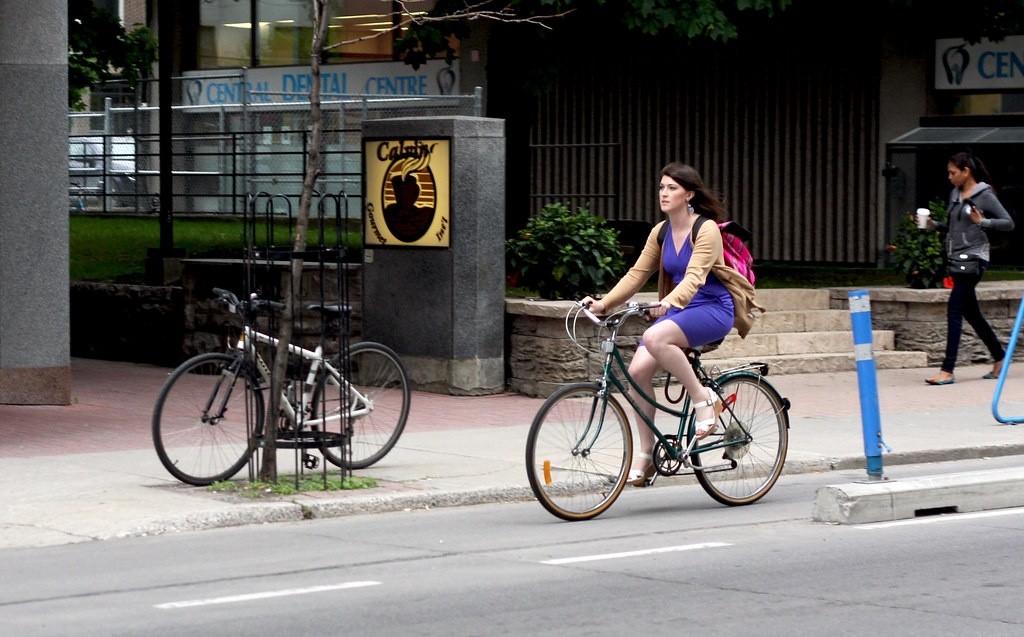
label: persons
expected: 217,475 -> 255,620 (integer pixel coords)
913,149 -> 1011,385
578,160 -> 736,487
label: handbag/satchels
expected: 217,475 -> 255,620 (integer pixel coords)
947,254 -> 981,279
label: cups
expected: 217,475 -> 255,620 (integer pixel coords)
917,208 -> 929,229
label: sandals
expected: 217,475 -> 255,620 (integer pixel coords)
692,387 -> 723,440
625,453 -> 656,485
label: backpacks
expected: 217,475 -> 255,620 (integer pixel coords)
656,214 -> 756,293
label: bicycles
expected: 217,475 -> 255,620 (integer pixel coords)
521,293 -> 793,525
72,181 -> 96,211
150,285 -> 413,488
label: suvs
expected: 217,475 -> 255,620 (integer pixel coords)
67,134 -> 137,209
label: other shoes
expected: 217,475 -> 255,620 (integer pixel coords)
924,371 -> 956,384
982,359 -> 1012,379
